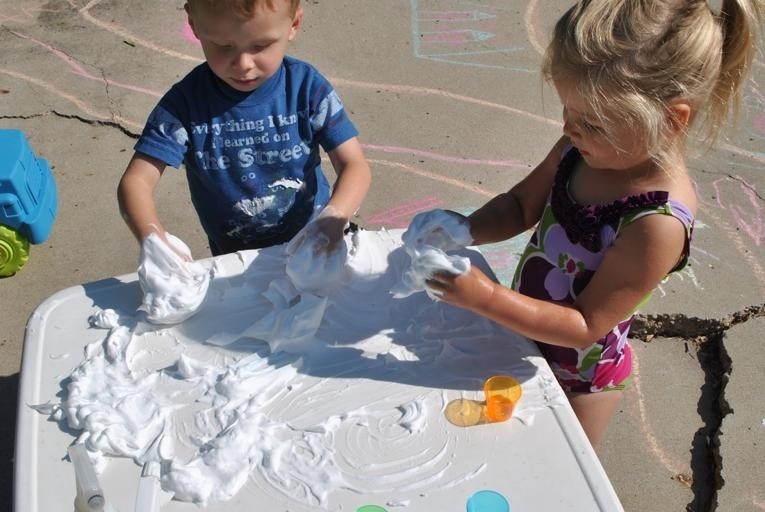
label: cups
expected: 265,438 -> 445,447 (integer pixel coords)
485,375 -> 521,422
466,490 -> 509,512
445,399 -> 489,428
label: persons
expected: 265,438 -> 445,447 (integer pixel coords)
402,0 -> 765,448
116,0 -> 373,310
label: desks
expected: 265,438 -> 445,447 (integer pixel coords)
11,228 -> 626,511
